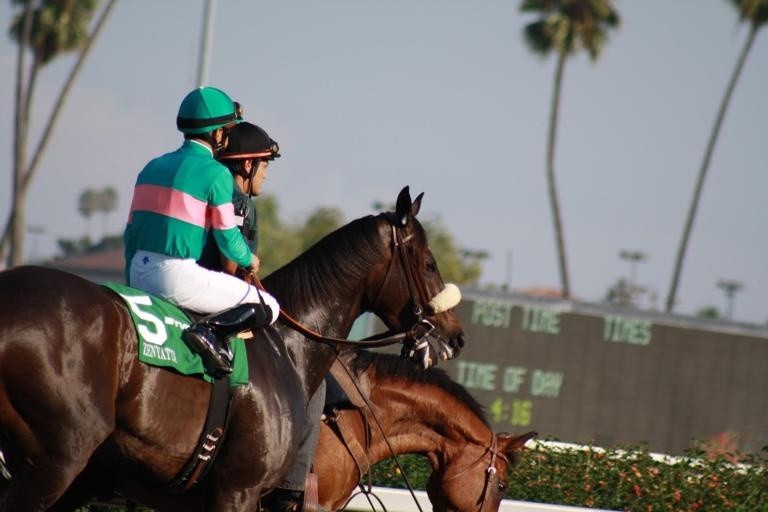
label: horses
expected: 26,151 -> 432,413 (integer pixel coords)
255,346 -> 539,512
0,185 -> 467,512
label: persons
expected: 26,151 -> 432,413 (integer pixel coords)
197,122 -> 280,270
120,86 -> 280,378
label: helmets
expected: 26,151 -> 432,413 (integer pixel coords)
221,122 -> 281,161
175,87 -> 245,134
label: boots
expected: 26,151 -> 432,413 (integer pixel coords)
183,302 -> 270,375
